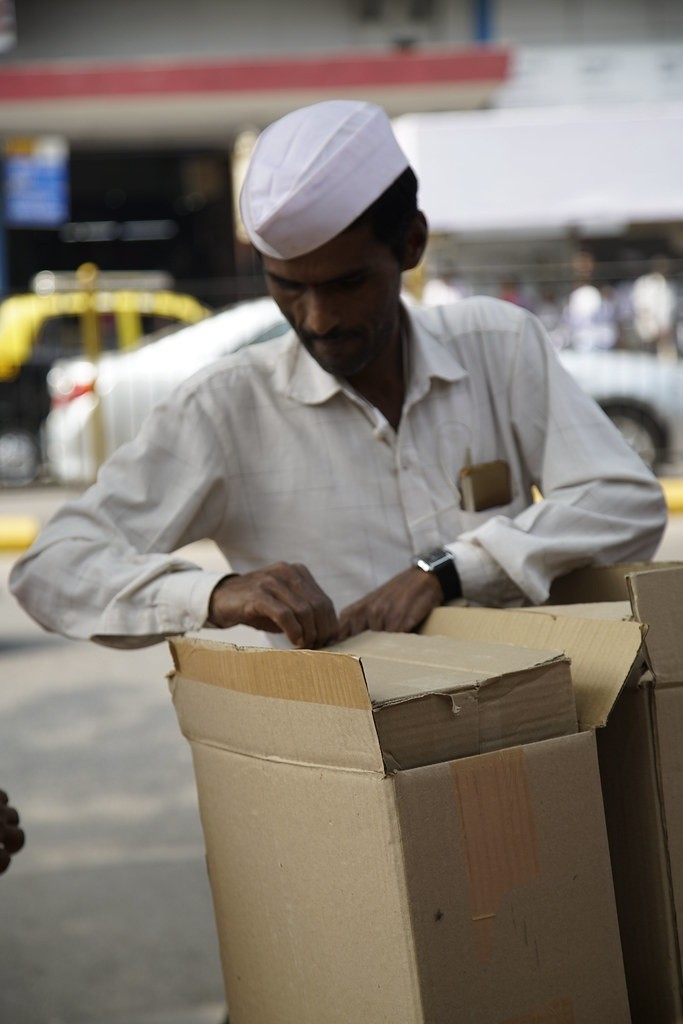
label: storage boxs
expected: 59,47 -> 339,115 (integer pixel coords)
164,559 -> 683,1024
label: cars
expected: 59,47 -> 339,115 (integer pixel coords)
45,295 -> 683,494
0,292 -> 214,489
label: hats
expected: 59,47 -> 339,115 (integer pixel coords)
240,99 -> 411,262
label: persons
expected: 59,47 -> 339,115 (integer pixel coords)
16,98 -> 668,653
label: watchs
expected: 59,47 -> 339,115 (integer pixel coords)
410,545 -> 463,607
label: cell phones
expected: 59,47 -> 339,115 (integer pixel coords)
460,458 -> 512,512
